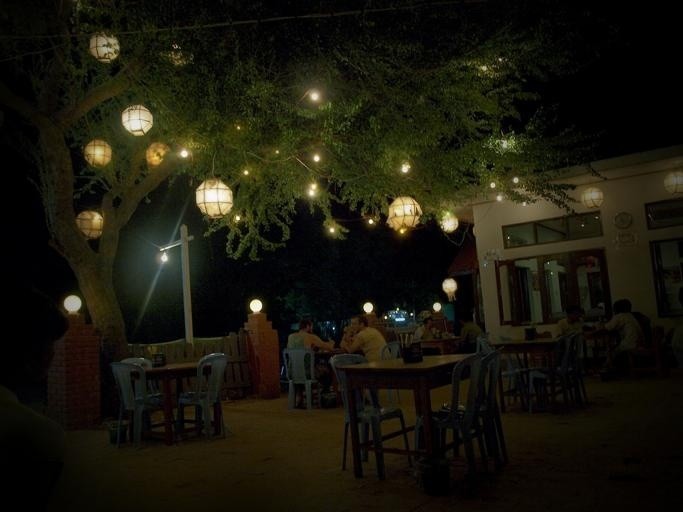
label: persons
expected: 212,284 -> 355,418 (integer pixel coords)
412,317 -> 437,342
338,326 -> 357,349
552,297 -> 653,381
456,310 -> 484,353
281,318 -> 335,409
342,315 -> 392,363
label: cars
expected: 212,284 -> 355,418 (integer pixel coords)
383,313 -> 408,328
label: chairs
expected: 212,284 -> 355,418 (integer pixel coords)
331,353 -> 412,480
412,349 -> 508,481
178,353 -> 228,441
484,325 -> 611,416
597,324 -> 683,381
283,325 -> 484,409
110,353 -> 164,448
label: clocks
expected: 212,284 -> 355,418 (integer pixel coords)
613,210 -> 634,229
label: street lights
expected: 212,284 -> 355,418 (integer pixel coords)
157,225 -> 195,343
